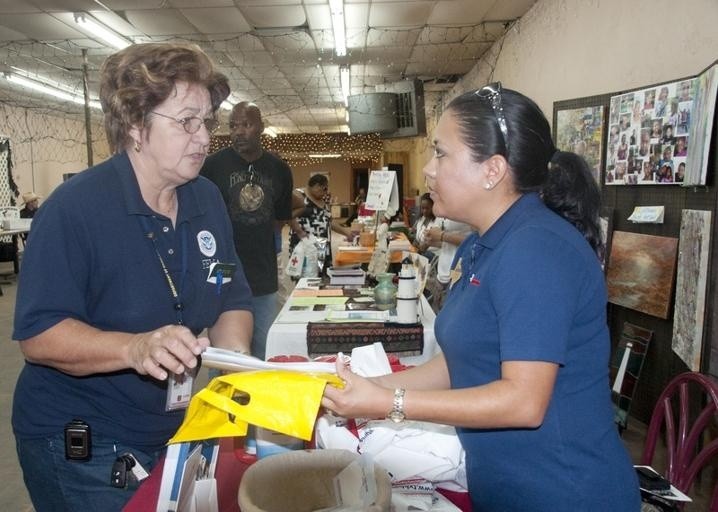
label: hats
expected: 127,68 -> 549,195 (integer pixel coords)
21,192 -> 43,206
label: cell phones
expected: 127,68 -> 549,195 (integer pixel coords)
65,422 -> 92,463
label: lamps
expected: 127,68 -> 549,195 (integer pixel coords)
327,0 -> 352,139
0,8 -> 280,139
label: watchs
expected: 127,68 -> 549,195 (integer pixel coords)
389,387 -> 405,423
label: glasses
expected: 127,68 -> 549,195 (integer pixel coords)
147,110 -> 220,134
475,81 -> 510,162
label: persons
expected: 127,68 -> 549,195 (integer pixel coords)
8,32 -> 253,512
322,80 -> 643,512
274,193 -> 305,253
288,160 -> 355,286
607,82 -> 695,183
424,215 -> 473,316
413,191 -> 439,263
20,191 -> 42,219
200,102 -> 294,381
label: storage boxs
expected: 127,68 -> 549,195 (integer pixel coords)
3,218 -> 32,230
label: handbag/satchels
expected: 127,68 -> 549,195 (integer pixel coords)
291,239 -> 318,280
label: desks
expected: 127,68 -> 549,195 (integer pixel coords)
0,229 -> 31,273
334,232 -> 417,268
330,202 -> 356,218
125,355 -> 473,512
265,277 -> 440,367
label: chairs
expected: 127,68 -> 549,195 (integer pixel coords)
633,370 -> 718,512
